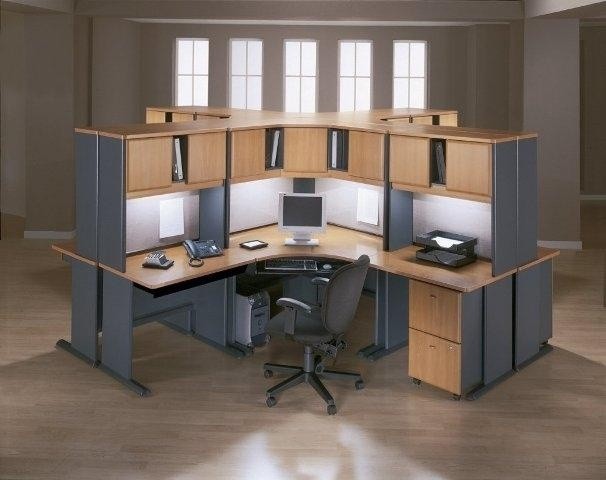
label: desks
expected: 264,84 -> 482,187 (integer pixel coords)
52,223 -> 561,402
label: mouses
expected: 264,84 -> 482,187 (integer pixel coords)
323,263 -> 332,270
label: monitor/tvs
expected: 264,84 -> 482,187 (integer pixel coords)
278,192 -> 326,246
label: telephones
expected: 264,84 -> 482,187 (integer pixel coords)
183,239 -> 225,267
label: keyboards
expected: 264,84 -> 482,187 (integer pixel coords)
265,259 -> 318,271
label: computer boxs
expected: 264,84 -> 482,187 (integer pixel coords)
235,281 -> 271,347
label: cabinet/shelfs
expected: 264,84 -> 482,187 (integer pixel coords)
75,107 -> 539,278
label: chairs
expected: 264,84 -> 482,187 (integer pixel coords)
262,254 -> 370,415
